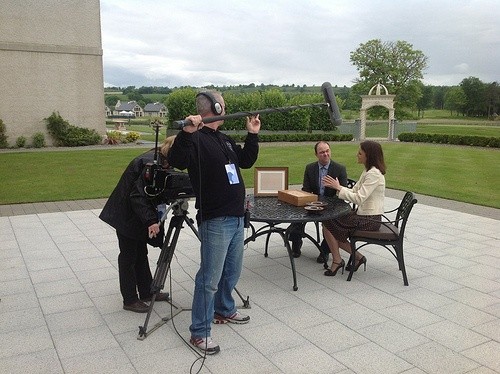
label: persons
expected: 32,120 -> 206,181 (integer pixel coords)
98,135 -> 177,313
291,141 -> 348,262
167,90 -> 260,354
323,141 -> 386,276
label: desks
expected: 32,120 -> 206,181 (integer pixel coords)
242,193 -> 351,292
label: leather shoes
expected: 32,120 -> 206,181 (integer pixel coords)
291,240 -> 302,257
141,291 -> 169,302
317,243 -> 329,262
123,300 -> 152,313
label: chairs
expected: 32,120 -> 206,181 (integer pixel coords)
285,179 -> 418,286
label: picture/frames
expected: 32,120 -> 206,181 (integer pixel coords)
253,167 -> 288,198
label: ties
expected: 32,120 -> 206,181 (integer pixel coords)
320,168 -> 327,195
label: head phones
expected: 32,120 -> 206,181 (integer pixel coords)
195,91 -> 222,116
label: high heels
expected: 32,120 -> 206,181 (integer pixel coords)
346,256 -> 366,272
324,259 -> 345,276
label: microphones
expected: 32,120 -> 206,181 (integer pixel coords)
321,81 -> 342,127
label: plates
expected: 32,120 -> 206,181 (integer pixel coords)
304,206 -> 324,210
306,201 -> 328,206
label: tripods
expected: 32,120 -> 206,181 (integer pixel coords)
136,198 -> 251,340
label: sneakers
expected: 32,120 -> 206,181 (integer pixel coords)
212,311 -> 250,325
189,336 -> 220,354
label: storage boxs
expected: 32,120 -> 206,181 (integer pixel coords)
277,190 -> 318,207
